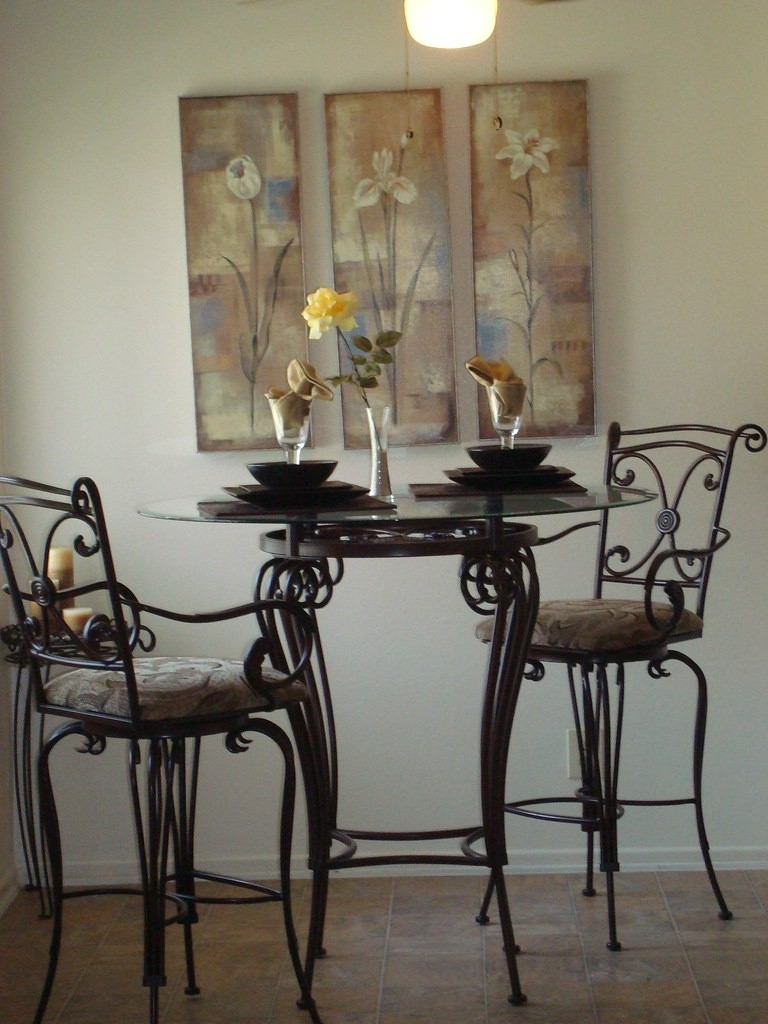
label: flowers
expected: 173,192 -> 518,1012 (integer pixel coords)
300,285 -> 402,406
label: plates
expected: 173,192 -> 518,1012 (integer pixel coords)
443,464 -> 577,488
222,481 -> 370,510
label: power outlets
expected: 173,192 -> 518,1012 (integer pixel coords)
567,728 -> 605,781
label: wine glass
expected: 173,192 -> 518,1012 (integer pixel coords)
485,387 -> 528,452
270,398 -> 312,465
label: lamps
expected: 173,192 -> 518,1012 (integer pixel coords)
403,0 -> 497,49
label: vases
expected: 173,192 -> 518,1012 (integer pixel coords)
364,408 -> 396,502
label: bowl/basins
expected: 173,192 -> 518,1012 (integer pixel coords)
245,459 -> 339,489
465,443 -> 552,472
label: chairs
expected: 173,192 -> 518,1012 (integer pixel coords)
0,473 -> 328,1024
475,419 -> 768,950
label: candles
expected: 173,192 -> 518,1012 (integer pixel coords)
27,577 -> 61,631
47,547 -> 76,607
63,606 -> 93,635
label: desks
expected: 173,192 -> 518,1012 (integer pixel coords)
4,620 -> 129,916
138,487 -> 658,1012
